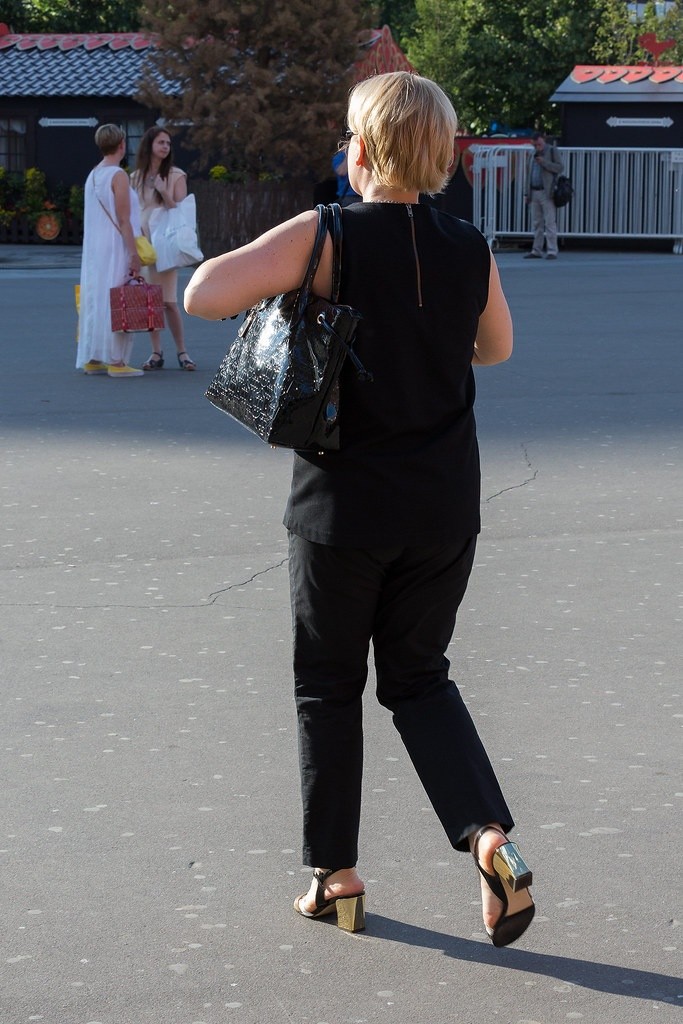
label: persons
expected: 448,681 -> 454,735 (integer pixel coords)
75,125 -> 196,376
524,134 -> 565,259
184,71 -> 537,947
332,150 -> 363,208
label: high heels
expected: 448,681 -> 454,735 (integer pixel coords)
472,820 -> 537,948
294,866 -> 366,934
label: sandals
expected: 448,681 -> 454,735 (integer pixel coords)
176,350 -> 197,370
140,349 -> 165,371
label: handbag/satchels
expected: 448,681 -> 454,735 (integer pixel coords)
552,175 -> 572,208
110,276 -> 165,331
204,203 -> 360,453
130,235 -> 158,267
147,192 -> 204,273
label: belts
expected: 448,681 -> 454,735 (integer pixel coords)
528,185 -> 543,190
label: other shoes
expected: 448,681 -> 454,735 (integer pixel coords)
545,254 -> 557,259
523,252 -> 542,258
84,361 -> 107,375
108,364 -> 144,377
